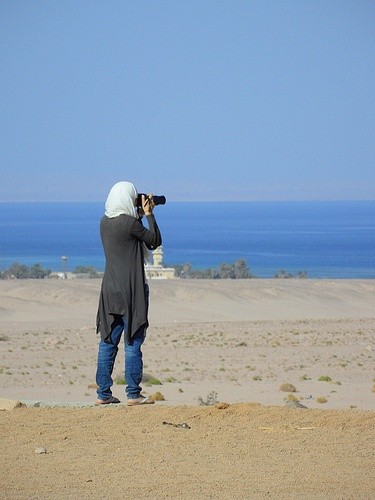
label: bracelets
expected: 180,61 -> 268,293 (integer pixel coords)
144,210 -> 152,213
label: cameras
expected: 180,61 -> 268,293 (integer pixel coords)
138,194 -> 166,207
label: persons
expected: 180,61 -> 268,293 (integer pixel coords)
92,180 -> 163,405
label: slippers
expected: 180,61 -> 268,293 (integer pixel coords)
127,397 -> 155,406
95,396 -> 120,406
